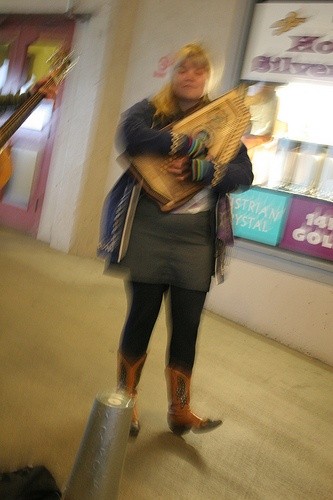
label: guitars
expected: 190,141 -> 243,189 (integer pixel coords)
0,45 -> 79,193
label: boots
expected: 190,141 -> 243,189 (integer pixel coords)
115,350 -> 147,436
163,366 -> 223,436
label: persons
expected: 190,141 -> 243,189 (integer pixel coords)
0,71 -> 61,112
93,44 -> 254,438
